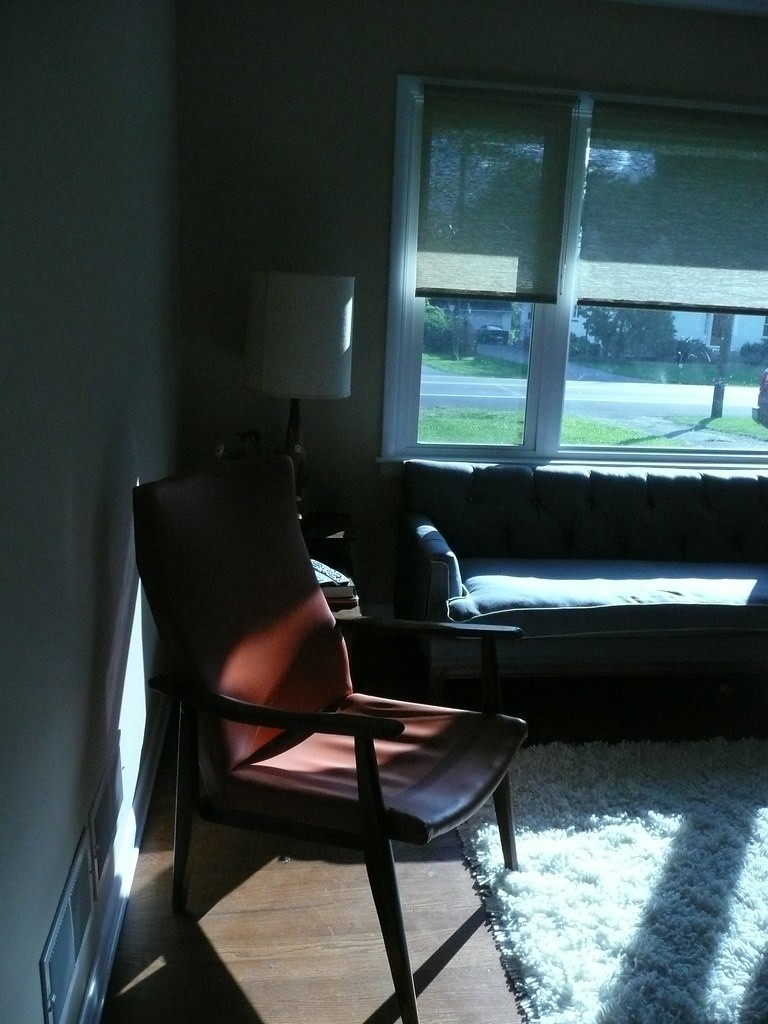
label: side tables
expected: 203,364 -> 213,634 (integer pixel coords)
301,511 -> 352,569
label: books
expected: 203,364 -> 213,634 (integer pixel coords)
309,557 -> 359,608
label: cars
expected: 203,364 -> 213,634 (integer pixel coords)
477,325 -> 509,345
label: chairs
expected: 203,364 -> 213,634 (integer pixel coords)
131,452 -> 527,1024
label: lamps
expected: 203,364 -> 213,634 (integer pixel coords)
241,269 -> 356,522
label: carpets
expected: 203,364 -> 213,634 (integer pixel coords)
456,734 -> 768,1024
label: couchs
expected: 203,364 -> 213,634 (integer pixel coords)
389,460 -> 768,707
